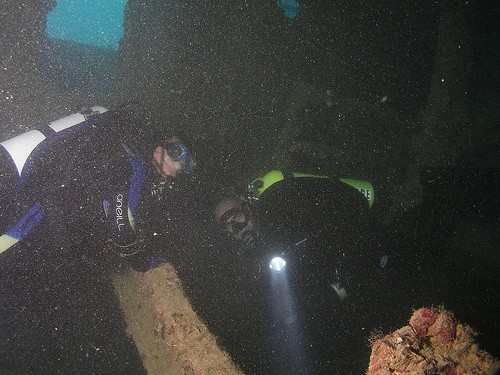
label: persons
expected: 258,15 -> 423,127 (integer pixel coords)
20,93 -> 201,274
208,159 -> 500,340
